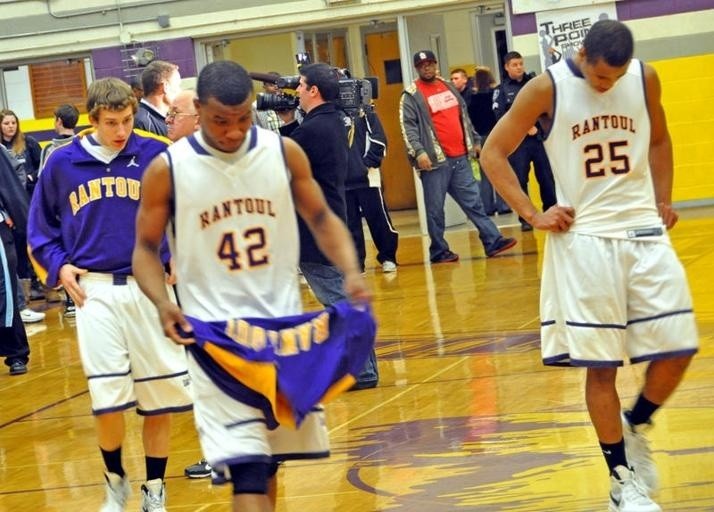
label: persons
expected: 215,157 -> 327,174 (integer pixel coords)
162,89 -> 230,485
128,62 -> 374,512
489,20 -> 700,512
25,78 -> 194,512
1,49 -> 559,373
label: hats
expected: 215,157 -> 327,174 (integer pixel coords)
414,50 -> 437,65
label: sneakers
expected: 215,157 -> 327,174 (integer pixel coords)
608,466 -> 664,511
382,260 -> 396,272
207,463 -> 232,484
9,360 -> 27,375
619,407 -> 661,491
488,237 -> 516,257
141,479 -> 169,512
522,222 -> 533,232
185,458 -> 210,478
97,478 -> 132,512
14,288 -> 76,323
431,249 -> 458,262
